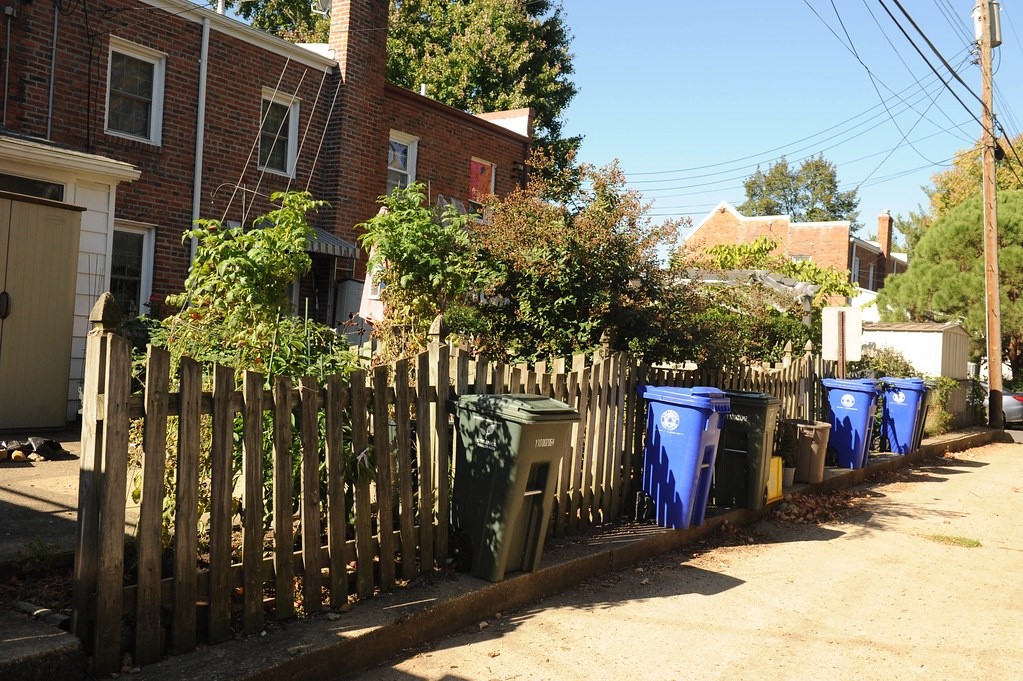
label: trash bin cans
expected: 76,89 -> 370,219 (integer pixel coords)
634,381 -> 732,530
819,378 -> 882,469
880,377 -> 933,454
783,419 -> 832,484
447,392 -> 582,582
715,389 -> 782,511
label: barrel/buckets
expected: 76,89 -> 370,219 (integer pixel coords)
783,467 -> 796,487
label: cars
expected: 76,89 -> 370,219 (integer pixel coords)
966,379 -> 1023,430
